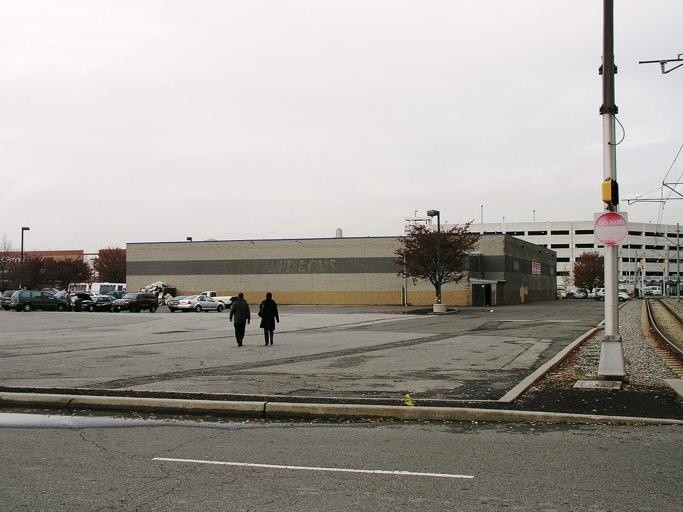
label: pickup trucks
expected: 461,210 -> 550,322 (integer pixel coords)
198,289 -> 238,309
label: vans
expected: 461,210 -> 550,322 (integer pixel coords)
643,285 -> 661,295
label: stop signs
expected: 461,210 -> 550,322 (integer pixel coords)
593,210 -> 629,246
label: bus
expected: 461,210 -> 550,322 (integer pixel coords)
66,281 -> 90,293
89,282 -> 125,293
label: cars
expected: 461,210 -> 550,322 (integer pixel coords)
0,287 -> 159,313
166,294 -> 188,313
555,284 -> 631,302
177,295 -> 226,313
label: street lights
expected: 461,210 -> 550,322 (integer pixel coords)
426,210 -> 446,313
19,226 -> 30,289
414,210 -> 417,224
186,237 -> 192,242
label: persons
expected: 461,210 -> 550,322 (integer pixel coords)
230,293 -> 250,346
260,292 -> 279,345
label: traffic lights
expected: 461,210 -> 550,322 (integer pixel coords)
601,177 -> 619,205
639,260 -> 644,268
662,262 -> 668,268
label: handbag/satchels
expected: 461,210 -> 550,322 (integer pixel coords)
258,309 -> 264,317
